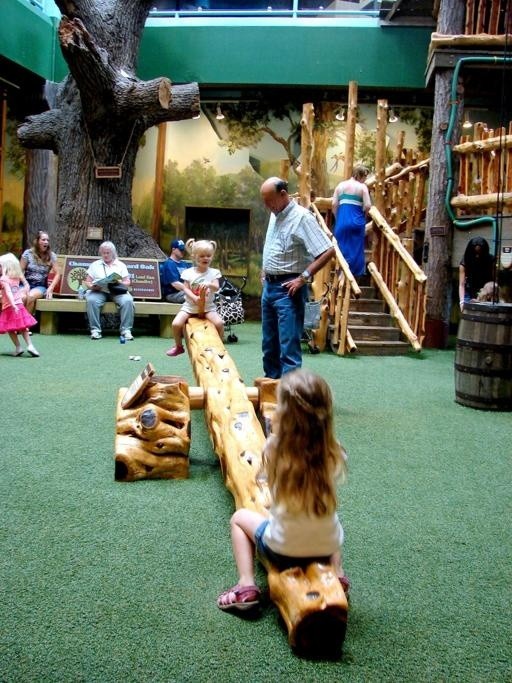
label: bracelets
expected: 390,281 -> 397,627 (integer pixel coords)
301,268 -> 311,280
459,299 -> 465,304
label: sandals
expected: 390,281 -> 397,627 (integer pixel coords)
334,577 -> 355,601
218,584 -> 267,610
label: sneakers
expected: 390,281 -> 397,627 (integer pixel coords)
166,345 -> 185,356
120,330 -> 135,341
25,346 -> 40,358
14,345 -> 24,357
90,332 -> 103,339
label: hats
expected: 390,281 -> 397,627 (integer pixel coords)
171,239 -> 186,250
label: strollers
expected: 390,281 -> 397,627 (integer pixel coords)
213,276 -> 249,345
295,281 -> 330,354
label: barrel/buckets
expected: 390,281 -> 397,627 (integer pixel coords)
453,301 -> 512,413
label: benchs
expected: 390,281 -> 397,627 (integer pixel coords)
35,299 -> 181,339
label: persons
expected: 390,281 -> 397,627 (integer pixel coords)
217,366 -> 350,618
456,234 -> 498,311
166,236 -> 225,356
83,239 -> 135,342
330,164 -> 370,281
1,251 -> 40,357
161,238 -> 187,303
259,175 -> 336,376
19,229 -> 61,335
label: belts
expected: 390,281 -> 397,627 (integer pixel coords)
264,273 -> 301,283
